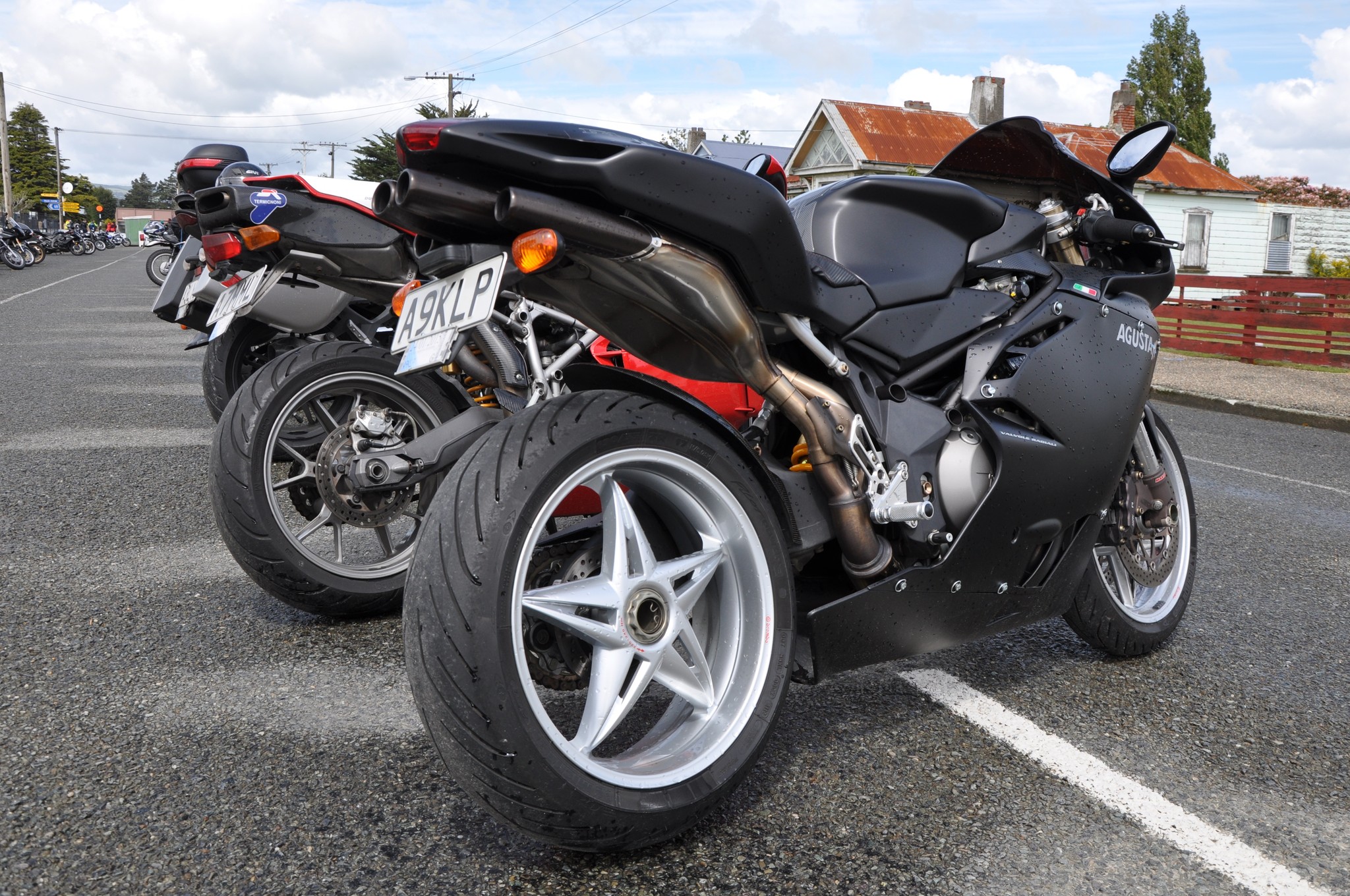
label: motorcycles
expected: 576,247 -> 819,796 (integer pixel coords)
144,220 -> 179,286
174,154 -> 790,618
146,195 -> 396,460
373,117 -> 1202,851
0,211 -> 131,270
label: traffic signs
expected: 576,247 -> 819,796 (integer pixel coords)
41,193 -> 79,213
